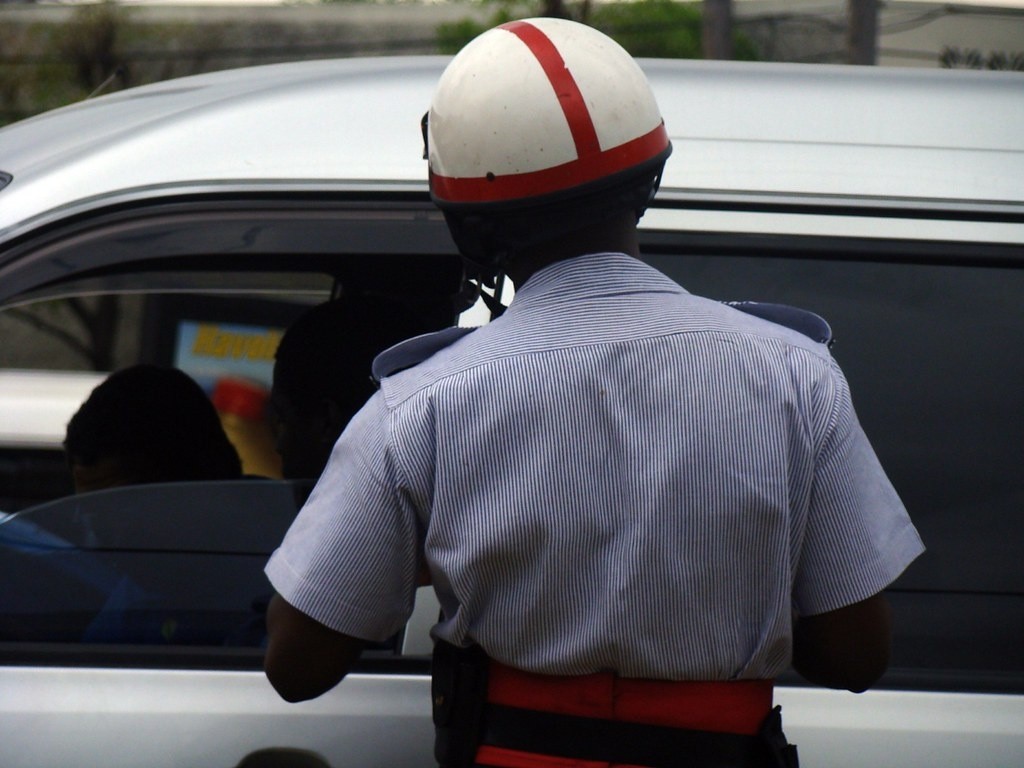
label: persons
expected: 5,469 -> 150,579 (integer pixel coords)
63,295 -> 431,647
262,16 -> 926,767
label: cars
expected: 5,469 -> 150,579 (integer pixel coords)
1,53 -> 1024,767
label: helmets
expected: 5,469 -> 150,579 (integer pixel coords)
421,18 -> 672,263
